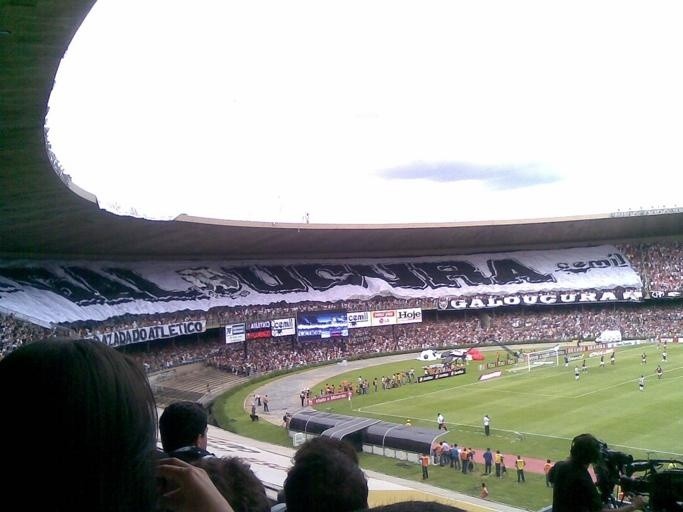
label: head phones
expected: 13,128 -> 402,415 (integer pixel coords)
570,433 -> 587,456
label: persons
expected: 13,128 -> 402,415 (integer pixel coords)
543,434 -> 683,511
419,413 -> 526,498
1,338 -> 464,510
202,239 -> 683,392
1,309 -> 201,359
